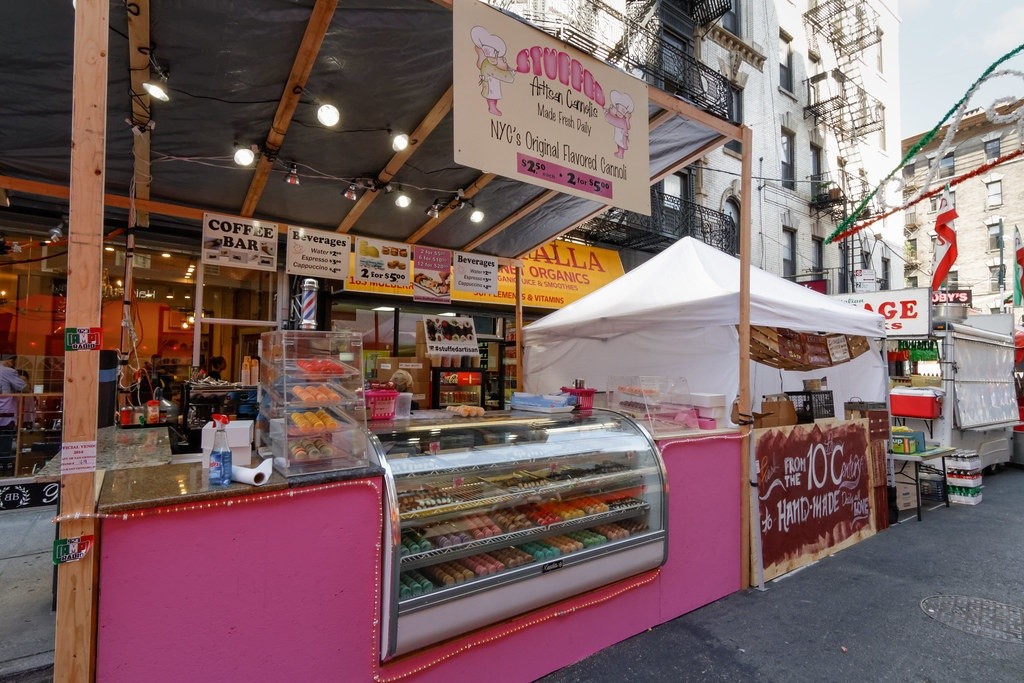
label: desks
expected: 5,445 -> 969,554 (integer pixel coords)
179,384 -> 257,436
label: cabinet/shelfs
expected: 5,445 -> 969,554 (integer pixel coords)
387,436 -> 664,609
259,333 -> 370,478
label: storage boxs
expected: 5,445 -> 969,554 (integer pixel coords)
844,399 -> 889,439
201,420 -> 254,448
947,493 -> 982,505
691,392 -> 727,430
804,378 -> 828,394
561,385 -> 596,410
365,321 -> 431,420
893,434 -> 915,454
754,399 -> 797,429
946,472 -> 982,489
890,386 -> 943,419
920,477 -> 945,501
203,445 -> 251,468
944,454 -> 981,471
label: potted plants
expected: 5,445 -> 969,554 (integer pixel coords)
811,181 -> 841,205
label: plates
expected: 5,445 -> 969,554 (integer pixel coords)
414,281 -> 450,297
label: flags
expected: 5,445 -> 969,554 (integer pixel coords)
930,184 -> 962,291
1012,224 -> 1024,309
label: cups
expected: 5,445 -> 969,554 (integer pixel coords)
431,353 -> 442,366
251,360 -> 258,385
242,362 -> 250,386
462,356 -> 472,368
229,415 -> 237,419
442,354 -> 451,367
451,355 -> 462,368
472,355 -> 481,368
244,356 -> 251,365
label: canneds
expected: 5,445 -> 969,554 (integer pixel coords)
440,392 -> 479,404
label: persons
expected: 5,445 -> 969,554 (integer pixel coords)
0,348 -> 36,478
134,352 -> 174,405
390,370 -> 420,410
204,356 -> 226,420
254,342 -> 348,448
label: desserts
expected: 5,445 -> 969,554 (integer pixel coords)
291,358 -> 346,459
398,460 -> 650,599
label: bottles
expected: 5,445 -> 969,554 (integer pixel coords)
133,406 -> 145,425
945,450 -> 982,497
898,340 -> 938,377
146,400 -> 160,423
121,406 -> 132,426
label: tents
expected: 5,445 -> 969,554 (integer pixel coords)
520,236 -> 898,593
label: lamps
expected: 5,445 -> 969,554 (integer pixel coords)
393,188 -> 411,210
424,196 -> 441,221
466,204 -> 485,223
142,52 -> 171,104
232,143 -> 254,166
282,164 -> 301,186
341,183 -> 357,202
387,129 -> 409,152
301,88 -> 340,127
